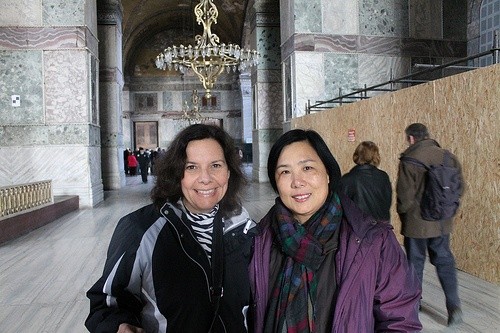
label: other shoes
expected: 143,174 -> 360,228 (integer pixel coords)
447,306 -> 464,327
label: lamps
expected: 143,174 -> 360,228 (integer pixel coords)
180,89 -> 201,120
155,0 -> 260,100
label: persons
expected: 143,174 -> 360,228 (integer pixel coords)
84,123 -> 257,333
395,123 -> 466,326
341,141 -> 392,223
246,129 -> 423,333
123,147 -> 167,185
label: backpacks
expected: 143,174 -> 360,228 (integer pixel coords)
400,150 -> 463,222
